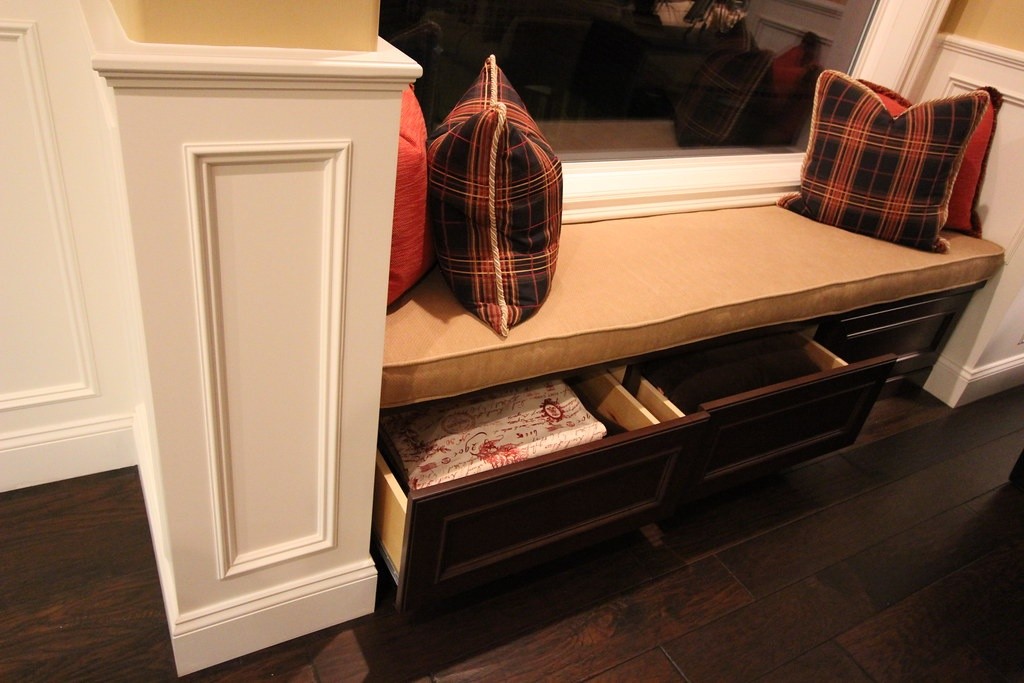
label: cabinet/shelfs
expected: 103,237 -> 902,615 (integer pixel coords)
370,205 -> 1005,621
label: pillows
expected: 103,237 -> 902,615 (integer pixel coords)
777,69 -> 990,255
671,38 -> 775,146
387,84 -> 437,306
856,78 -> 1004,240
762,33 -> 824,147
425,54 -> 563,338
